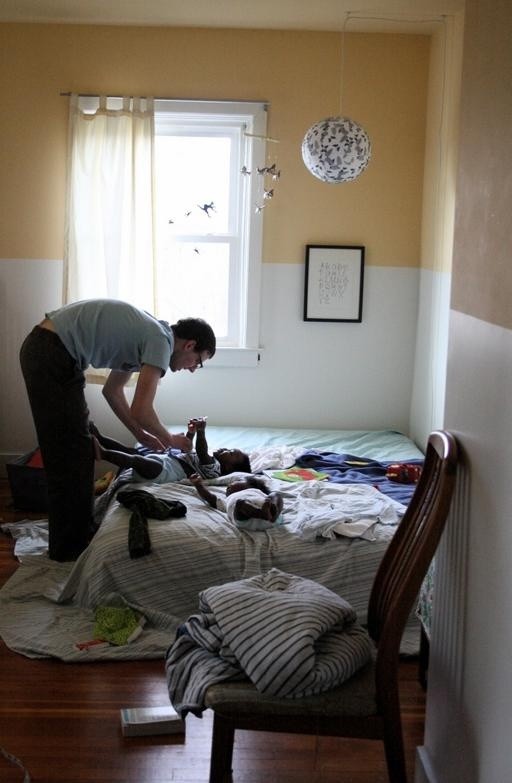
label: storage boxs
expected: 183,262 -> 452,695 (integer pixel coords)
4,447 -> 45,514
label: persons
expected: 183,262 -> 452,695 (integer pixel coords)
192,468 -> 284,533
16,291 -> 215,562
85,412 -> 254,486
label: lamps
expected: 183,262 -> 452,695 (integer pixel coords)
298,15 -> 372,186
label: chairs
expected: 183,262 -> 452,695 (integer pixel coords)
203,429 -> 459,783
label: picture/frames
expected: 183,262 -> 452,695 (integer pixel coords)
302,243 -> 366,323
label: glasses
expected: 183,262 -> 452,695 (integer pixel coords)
195,354 -> 203,369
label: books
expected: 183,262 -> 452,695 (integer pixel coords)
121,705 -> 188,737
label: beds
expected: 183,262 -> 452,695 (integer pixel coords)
88,423 -> 430,615
